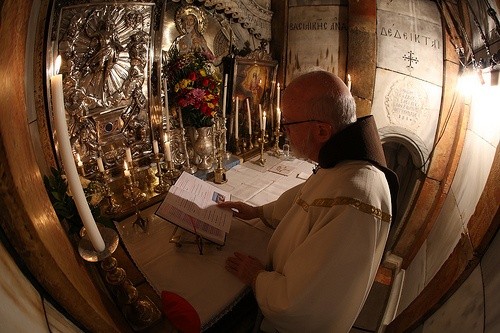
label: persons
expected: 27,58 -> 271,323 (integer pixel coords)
60,5 -> 153,166
216,70 -> 392,333
179,14 -> 211,55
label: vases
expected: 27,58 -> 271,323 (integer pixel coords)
194,127 -> 215,169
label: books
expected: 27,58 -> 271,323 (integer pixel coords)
155,171 -> 233,246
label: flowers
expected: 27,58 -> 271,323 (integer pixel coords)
164,50 -> 223,125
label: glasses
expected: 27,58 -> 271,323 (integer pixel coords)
280,118 -> 323,131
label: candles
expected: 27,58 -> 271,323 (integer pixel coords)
123,162 -> 129,174
153,141 -> 159,154
235,96 -> 239,139
126,148 -> 132,159
259,104 -> 267,130
276,82 -> 282,123
348,73 -> 351,91
222,74 -> 229,117
50,56 -> 105,252
165,142 -> 171,161
246,98 -> 252,134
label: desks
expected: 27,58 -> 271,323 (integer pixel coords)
115,146 -> 319,333
